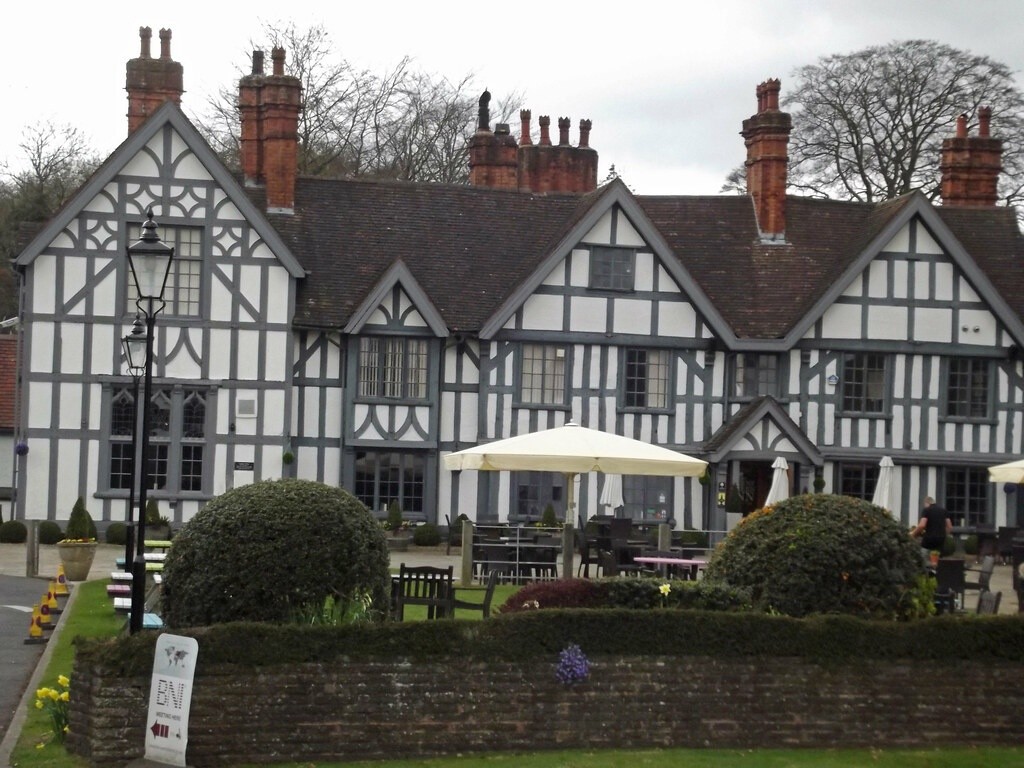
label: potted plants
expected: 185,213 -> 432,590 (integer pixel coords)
56,538 -> 97,581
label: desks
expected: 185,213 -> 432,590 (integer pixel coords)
145,563 -> 163,571
390,575 -> 459,622
143,541 -> 171,553
153,575 -> 161,583
143,553 -> 166,563
633,557 -> 704,581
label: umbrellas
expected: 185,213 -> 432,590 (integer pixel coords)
872,456 -> 895,512
445,419 -> 709,521
987,459 -> 1024,483
762,457 -> 789,507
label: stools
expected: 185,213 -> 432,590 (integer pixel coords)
106,584 -> 130,598
115,558 -> 124,569
110,573 -> 132,585
113,597 -> 131,615
128,613 -> 162,630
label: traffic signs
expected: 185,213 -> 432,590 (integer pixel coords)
142,631 -> 200,768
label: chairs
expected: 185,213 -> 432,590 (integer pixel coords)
443,515 -> 706,589
905,524 -> 1023,615
448,569 -> 498,619
393,563 -> 452,622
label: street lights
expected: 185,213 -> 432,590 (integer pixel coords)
121,301 -> 155,572
124,204 -> 175,637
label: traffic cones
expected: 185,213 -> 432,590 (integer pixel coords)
39,594 -> 56,630
24,603 -> 49,644
48,580 -> 58,608
55,562 -> 70,597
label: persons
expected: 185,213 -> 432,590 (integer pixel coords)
911,497 -> 953,566
